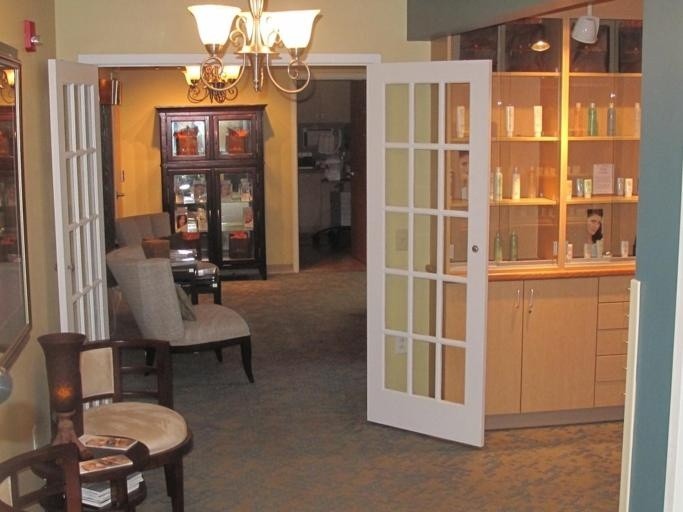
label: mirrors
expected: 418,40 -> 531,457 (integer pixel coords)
0,54 -> 35,379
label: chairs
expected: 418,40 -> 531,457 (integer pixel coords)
104,211 -> 255,385
0,332 -> 193,511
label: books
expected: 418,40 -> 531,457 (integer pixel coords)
74,432 -> 144,508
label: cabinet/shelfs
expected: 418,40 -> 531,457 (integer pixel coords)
154,103 -> 267,281
0,106 -> 18,261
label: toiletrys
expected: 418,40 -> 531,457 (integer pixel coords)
452,98 -> 639,264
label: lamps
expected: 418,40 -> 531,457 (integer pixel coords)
0,68 -> 15,106
570,2 -> 600,45
530,17 -> 551,53
179,0 -> 320,104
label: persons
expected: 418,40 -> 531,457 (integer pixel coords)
176,215 -> 187,228
